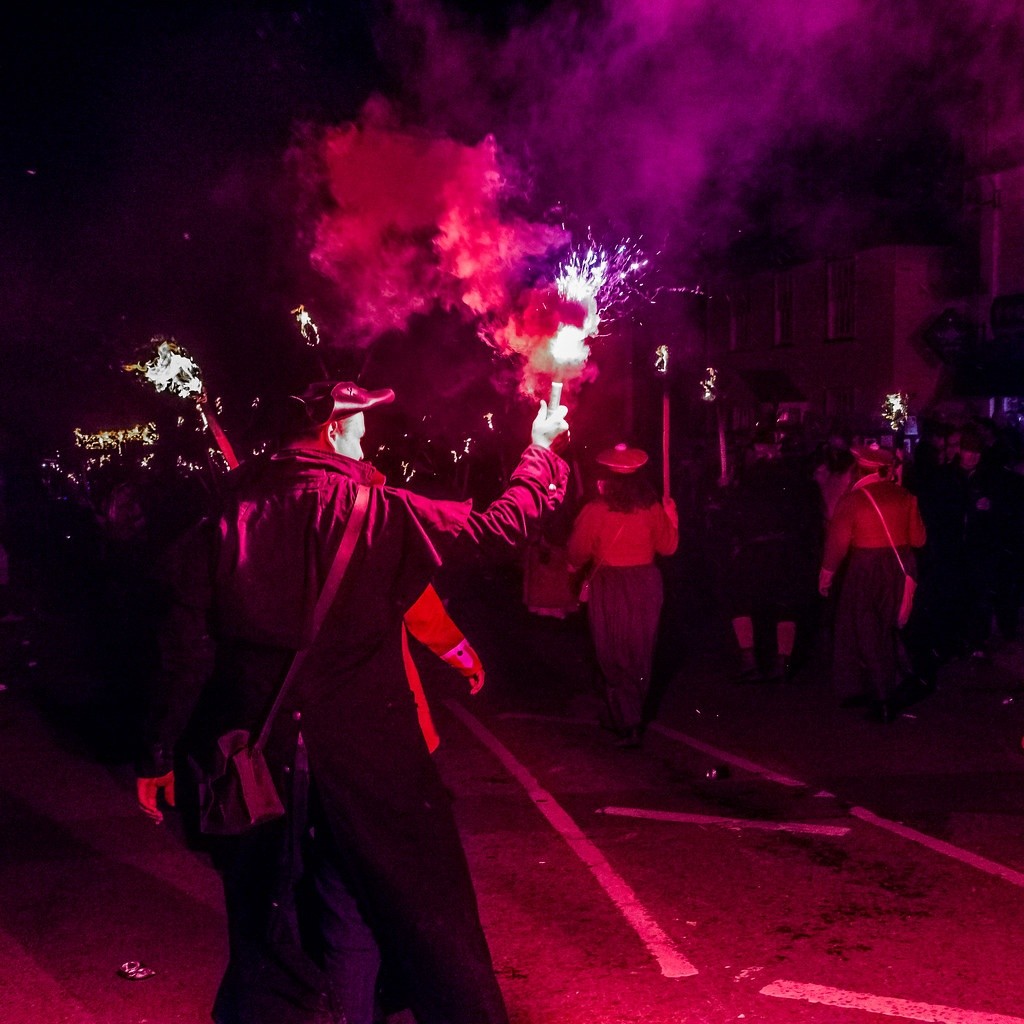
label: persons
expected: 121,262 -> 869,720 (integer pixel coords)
817,447 -> 927,724
565,447 -> 680,748
919,417 -> 1024,666
402,583 -> 485,754
708,431 -> 827,685
137,349 -> 572,1023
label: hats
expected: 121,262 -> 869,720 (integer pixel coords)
849,442 -> 896,472
261,371 -> 396,435
593,440 -> 650,476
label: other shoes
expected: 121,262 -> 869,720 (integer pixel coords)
601,716 -> 645,755
863,700 -> 891,723
775,658 -> 792,675
720,656 -> 759,680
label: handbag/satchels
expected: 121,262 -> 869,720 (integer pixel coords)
897,576 -> 918,630
194,729 -> 287,834
